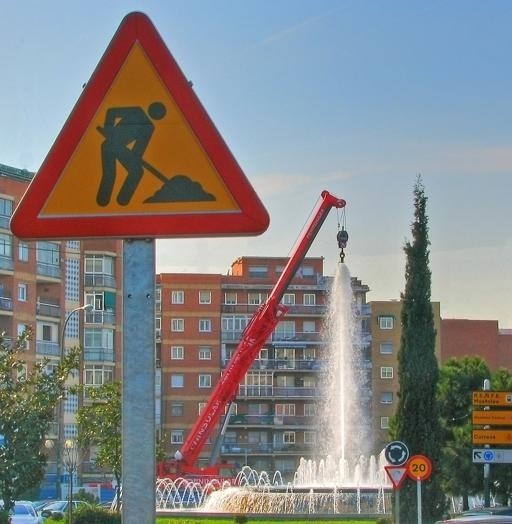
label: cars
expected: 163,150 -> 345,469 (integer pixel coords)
0,500 -> 93,524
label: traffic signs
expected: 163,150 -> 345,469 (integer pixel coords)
472,390 -> 512,464
407,456 -> 432,480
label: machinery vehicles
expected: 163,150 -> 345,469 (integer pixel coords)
156,190 -> 348,496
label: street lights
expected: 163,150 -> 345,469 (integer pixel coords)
45,440 -> 73,501
